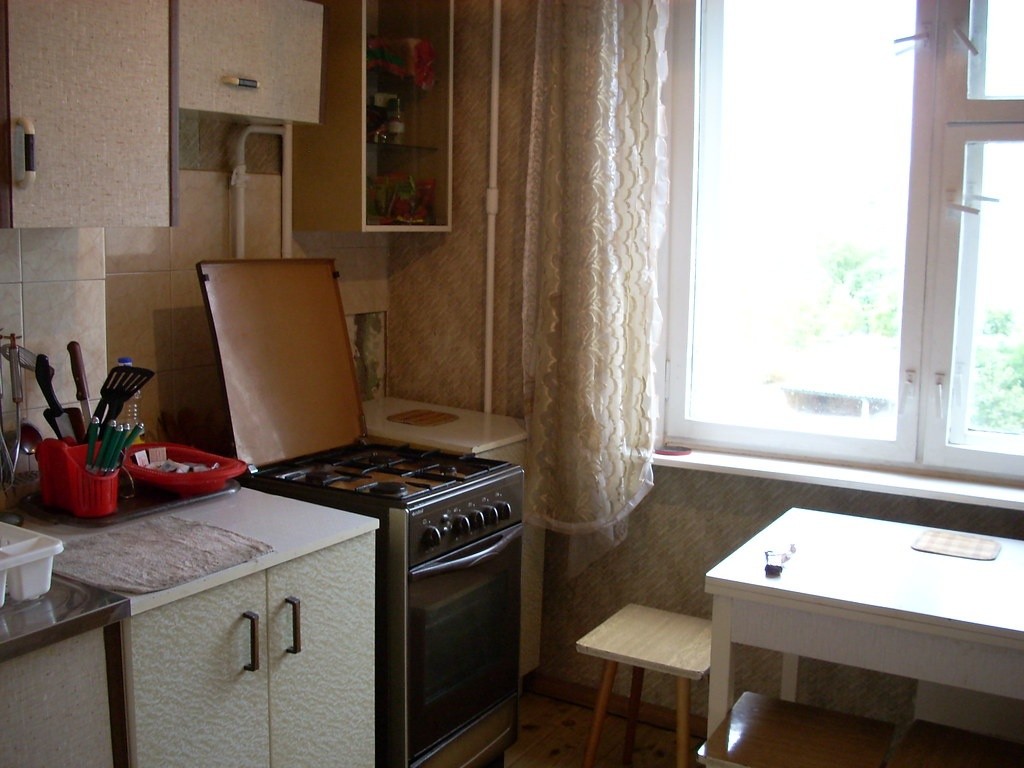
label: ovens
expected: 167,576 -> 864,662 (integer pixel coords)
371,469 -> 525,768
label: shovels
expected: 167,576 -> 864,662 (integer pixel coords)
98,367 -> 155,439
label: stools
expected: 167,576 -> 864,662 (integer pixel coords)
576,603 -> 711,768
698,691 -> 896,767
887,719 -> 1024,767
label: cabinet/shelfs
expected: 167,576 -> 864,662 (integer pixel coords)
293,0 -> 454,234
122,531 -> 375,768
0,627 -> 114,768
0,0 -> 180,228
181,0 -> 326,125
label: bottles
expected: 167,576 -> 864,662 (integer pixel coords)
118,357 -> 141,445
386,96 -> 407,144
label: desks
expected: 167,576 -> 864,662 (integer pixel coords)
705,508 -> 1023,768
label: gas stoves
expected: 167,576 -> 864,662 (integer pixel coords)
253,439 -> 512,504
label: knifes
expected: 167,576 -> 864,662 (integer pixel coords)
35,341 -> 93,445
